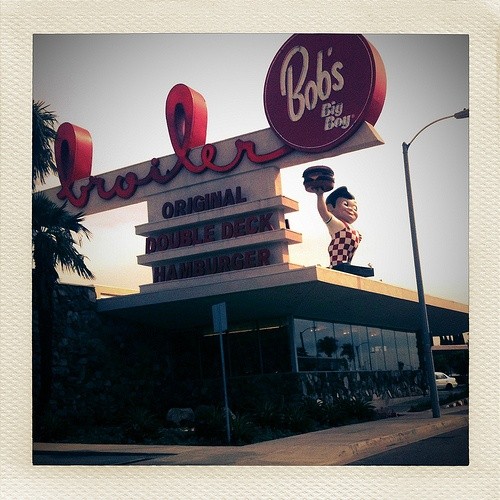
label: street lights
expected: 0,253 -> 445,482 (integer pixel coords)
401,108 -> 469,420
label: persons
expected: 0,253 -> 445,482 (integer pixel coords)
314,184 -> 362,270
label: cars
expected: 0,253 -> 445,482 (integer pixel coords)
434,372 -> 457,390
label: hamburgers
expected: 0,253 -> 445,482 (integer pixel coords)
302,165 -> 335,193
